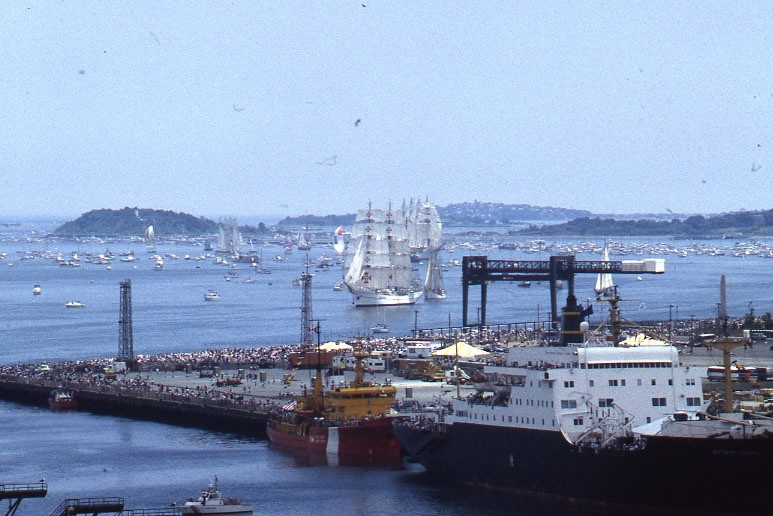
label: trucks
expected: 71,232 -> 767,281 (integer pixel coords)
103,362 -> 126,374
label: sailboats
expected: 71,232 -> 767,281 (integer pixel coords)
343,198 -> 425,306
594,239 -> 613,292
400,196 -> 446,262
422,242 -> 448,299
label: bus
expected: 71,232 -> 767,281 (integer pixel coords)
707,365 -> 773,381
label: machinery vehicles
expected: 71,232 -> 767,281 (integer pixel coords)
402,362 -> 471,385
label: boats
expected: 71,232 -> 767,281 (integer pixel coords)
176,474 -> 253,515
1,226 -> 411,291
33,287 -> 41,294
440,236 -> 604,272
204,290 -> 220,300
48,388 -> 80,410
385,286 -> 773,516
608,238 -> 773,258
265,331 -> 401,467
65,300 -> 85,307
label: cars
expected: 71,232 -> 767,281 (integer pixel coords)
484,345 -> 509,352
40,365 -> 50,371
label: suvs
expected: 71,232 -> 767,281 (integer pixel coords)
216,377 -> 241,387
